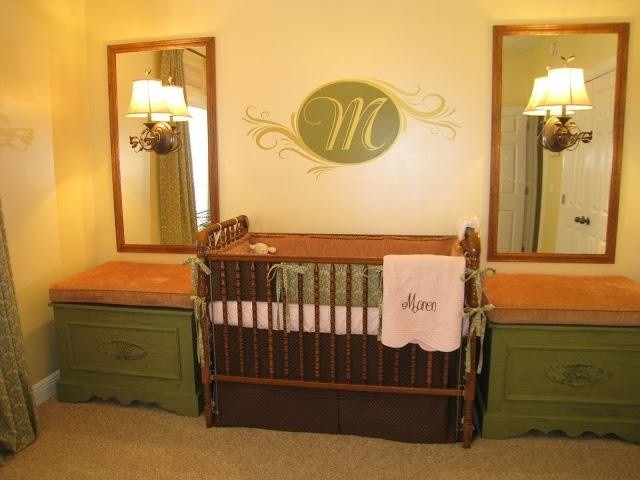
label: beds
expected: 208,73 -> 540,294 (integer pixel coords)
200,212 -> 479,449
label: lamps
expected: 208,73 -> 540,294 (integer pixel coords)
125,70 -> 192,154
521,56 -> 592,152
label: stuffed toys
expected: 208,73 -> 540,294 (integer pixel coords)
249,243 -> 276,255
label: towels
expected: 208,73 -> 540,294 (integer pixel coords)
381,255 -> 465,353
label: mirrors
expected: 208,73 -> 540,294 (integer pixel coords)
486,22 -> 629,265
107,35 -> 221,255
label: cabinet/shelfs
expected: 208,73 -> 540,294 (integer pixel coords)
49,303 -> 206,419
476,322 -> 639,445
